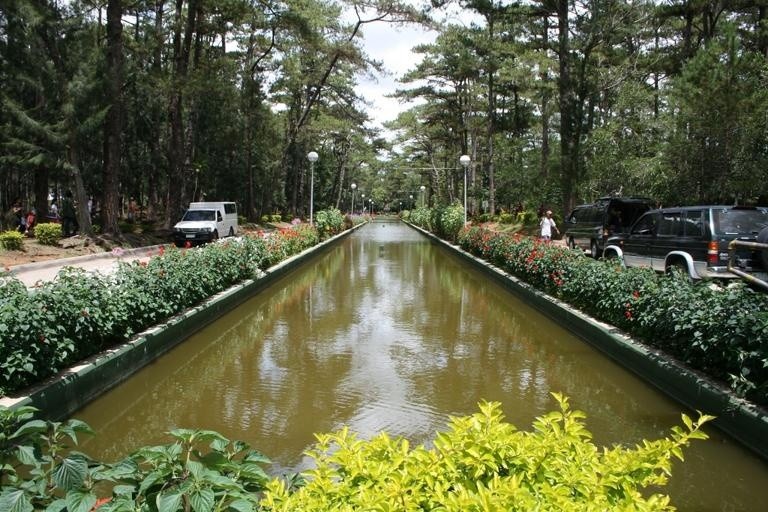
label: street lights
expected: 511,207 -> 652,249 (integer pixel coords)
308,151 -> 318,227
361,193 -> 374,218
399,202 -> 402,212
420,185 -> 426,229
459,153 -> 472,231
409,194 -> 414,217
350,183 -> 356,219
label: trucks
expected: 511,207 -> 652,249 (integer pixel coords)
173,201 -> 239,245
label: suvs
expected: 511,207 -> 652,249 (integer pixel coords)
603,196 -> 768,287
564,192 -> 659,263
724,223 -> 768,298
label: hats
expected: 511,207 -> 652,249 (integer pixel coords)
546,211 -> 552,214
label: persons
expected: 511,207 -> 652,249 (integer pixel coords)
49,199 -> 60,223
539,210 -> 561,240
8,199 -> 24,231
63,189 -> 80,237
24,210 -> 37,236
127,197 -> 137,225
